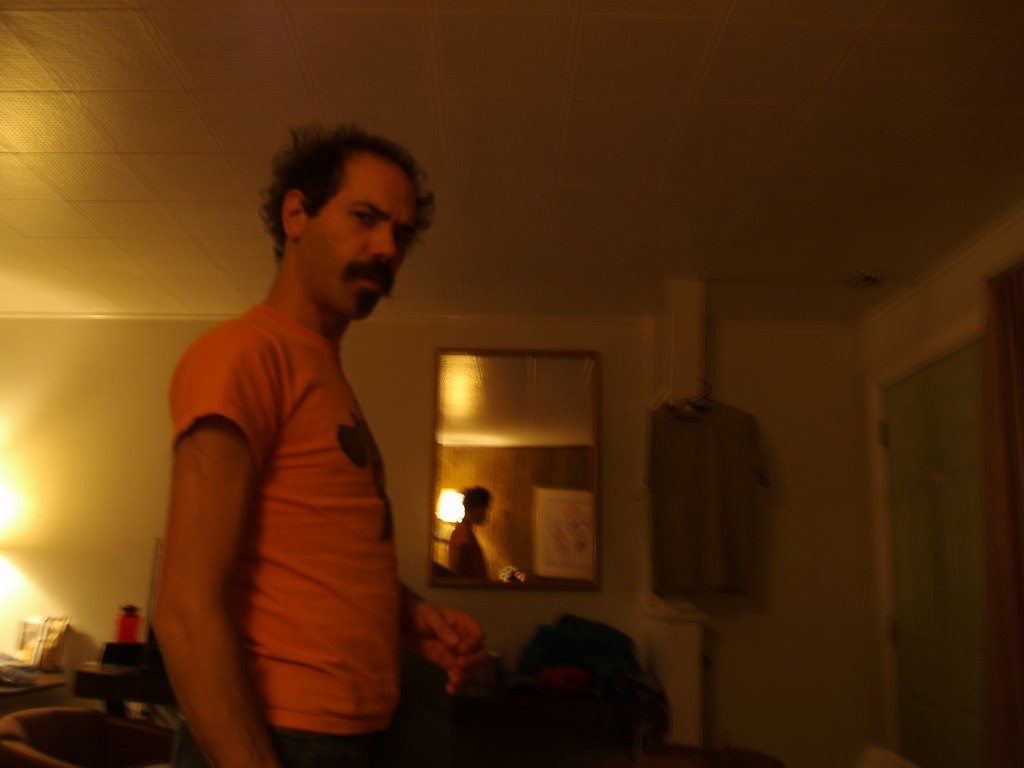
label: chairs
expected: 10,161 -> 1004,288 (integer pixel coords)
0,706 -> 176,768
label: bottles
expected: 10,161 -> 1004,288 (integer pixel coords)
113,605 -> 145,642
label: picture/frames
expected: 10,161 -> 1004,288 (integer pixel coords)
427,346 -> 602,592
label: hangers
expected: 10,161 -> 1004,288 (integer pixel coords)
670,377 -> 716,419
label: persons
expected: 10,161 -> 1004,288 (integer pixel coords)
449,485 -> 489,579
153,126 -> 482,768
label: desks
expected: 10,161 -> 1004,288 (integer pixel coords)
72,665 -> 141,720
1,670 -> 69,695
